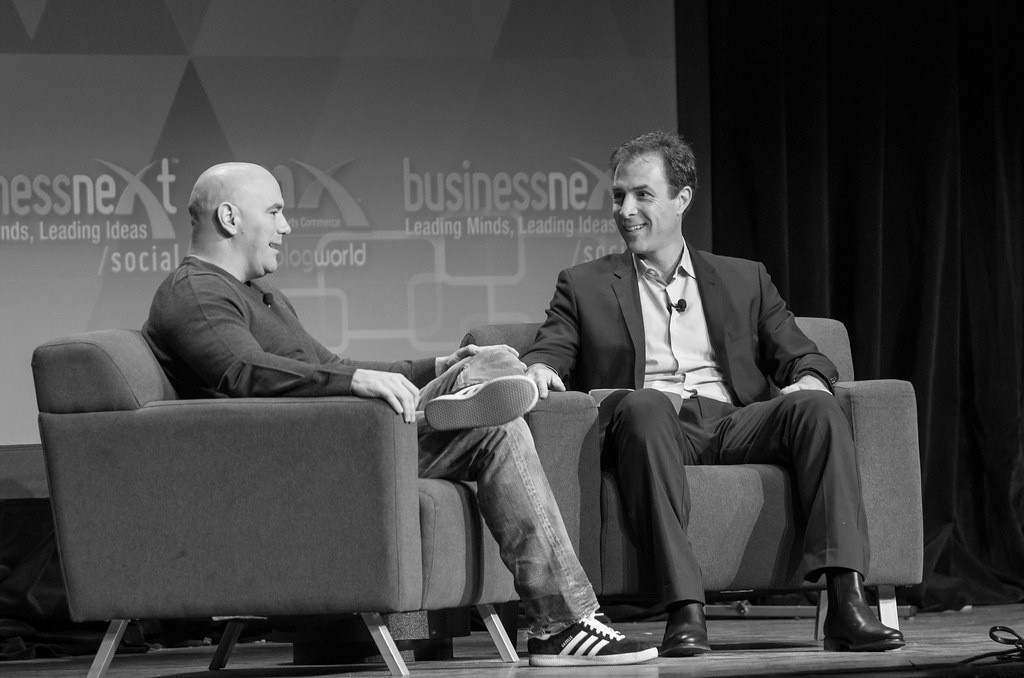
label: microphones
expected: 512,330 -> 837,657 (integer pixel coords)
263,292 -> 273,306
677,299 -> 687,312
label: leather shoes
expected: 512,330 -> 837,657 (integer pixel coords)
661,598 -> 711,656
822,567 -> 905,653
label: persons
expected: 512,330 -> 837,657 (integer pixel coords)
141,161 -> 658,667
518,130 -> 905,657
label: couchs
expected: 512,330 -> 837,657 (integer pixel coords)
30,327 -> 600,678
458,312 -> 925,644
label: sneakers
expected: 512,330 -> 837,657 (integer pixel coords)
424,376 -> 537,430
527,609 -> 659,667
